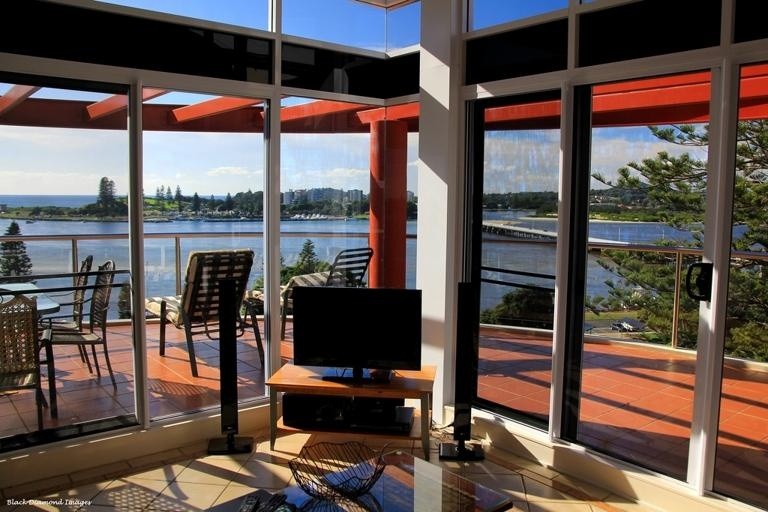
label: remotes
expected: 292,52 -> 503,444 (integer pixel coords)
237,492 -> 260,512
257,493 -> 289,512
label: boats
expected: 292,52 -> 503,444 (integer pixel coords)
26,220 -> 34,224
289,213 -> 328,221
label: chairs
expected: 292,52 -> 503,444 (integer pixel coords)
40,259 -> 120,394
244,245 -> 375,341
0,289 -> 60,435
137,248 -> 265,377
29,254 -> 92,375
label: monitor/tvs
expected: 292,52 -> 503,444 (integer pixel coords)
292,286 -> 422,387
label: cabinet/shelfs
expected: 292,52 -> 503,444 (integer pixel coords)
264,362 -> 437,458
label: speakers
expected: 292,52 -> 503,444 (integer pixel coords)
208,277 -> 252,454
438,283 -> 487,460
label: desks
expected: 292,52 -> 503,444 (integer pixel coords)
1,278 -> 60,317
